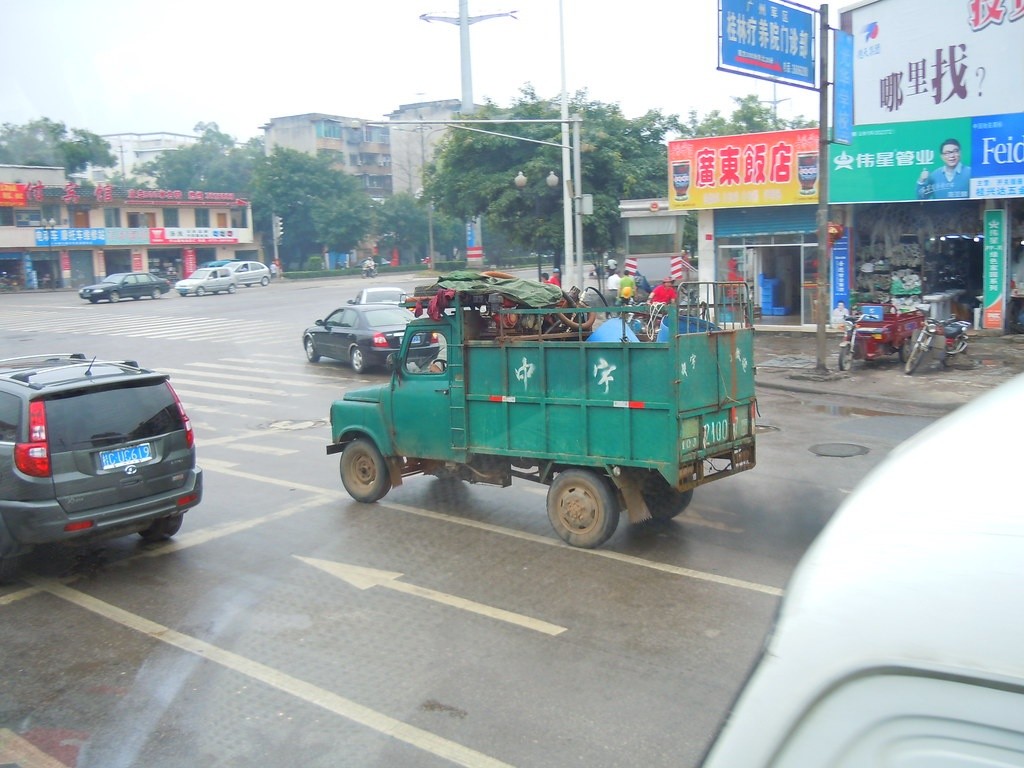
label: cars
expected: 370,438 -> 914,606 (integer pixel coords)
196,260 -> 230,269
346,287 -> 414,311
174,267 -> 238,297
302,304 -> 439,374
78,273 -> 171,303
222,262 -> 271,286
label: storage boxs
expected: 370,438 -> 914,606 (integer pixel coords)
758,274 -> 791,316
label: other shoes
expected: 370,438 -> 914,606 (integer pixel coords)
606,317 -> 611,319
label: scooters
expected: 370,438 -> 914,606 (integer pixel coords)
361,263 -> 378,279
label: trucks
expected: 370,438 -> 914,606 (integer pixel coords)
325,271 -> 761,548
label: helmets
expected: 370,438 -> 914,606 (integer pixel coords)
553,268 -> 559,273
622,287 -> 632,299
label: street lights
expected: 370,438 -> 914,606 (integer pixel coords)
514,171 -> 559,282
40,217 -> 57,290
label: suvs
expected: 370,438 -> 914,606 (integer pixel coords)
0,353 -> 205,589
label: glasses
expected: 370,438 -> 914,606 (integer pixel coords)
942,148 -> 960,156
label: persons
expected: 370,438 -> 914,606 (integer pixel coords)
833,301 -> 848,323
607,257 -> 617,276
270,261 -> 276,279
365,257 -> 374,277
430,364 -> 442,372
550,268 -> 560,287
646,276 -> 677,305
540,272 -> 552,285
607,269 -> 621,306
617,270 -> 637,306
915,138 -> 970,200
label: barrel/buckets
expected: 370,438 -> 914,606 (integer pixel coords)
656,314 -> 723,342
583,317 -> 641,342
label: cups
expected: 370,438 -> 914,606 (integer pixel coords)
797,151 -> 818,196
671,160 -> 691,201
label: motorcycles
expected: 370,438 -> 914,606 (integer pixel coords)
904,313 -> 972,375
838,303 -> 925,371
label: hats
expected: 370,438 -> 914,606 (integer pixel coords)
663,277 -> 675,284
